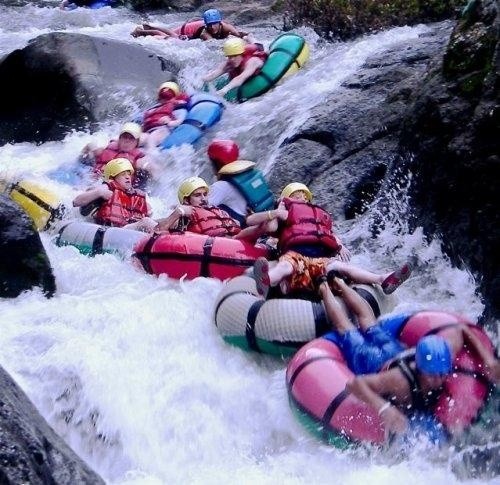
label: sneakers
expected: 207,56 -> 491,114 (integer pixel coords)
253,257 -> 271,295
327,270 -> 343,296
313,275 -> 327,301
381,262 -> 414,295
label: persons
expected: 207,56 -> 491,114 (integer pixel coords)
110,80 -> 190,151
58,0 -> 96,15
72,157 -> 159,234
206,139 -> 279,230
200,38 -> 269,98
154,176 -> 286,248
129,7 -> 249,41
76,121 -> 153,189
311,269 -> 500,437
245,180 -> 415,299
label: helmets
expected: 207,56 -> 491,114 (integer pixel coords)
415,335 -> 450,373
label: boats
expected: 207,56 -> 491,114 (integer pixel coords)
122,91 -> 225,153
63,0 -> 119,12
0,168 -> 70,234
203,33 -> 309,106
50,210 -> 167,261
132,224 -> 282,285
212,257 -> 390,357
153,20 -> 241,41
285,310 -> 500,460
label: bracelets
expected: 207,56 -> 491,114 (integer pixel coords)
177,207 -> 185,217
266,210 -> 273,221
377,401 -> 390,420
